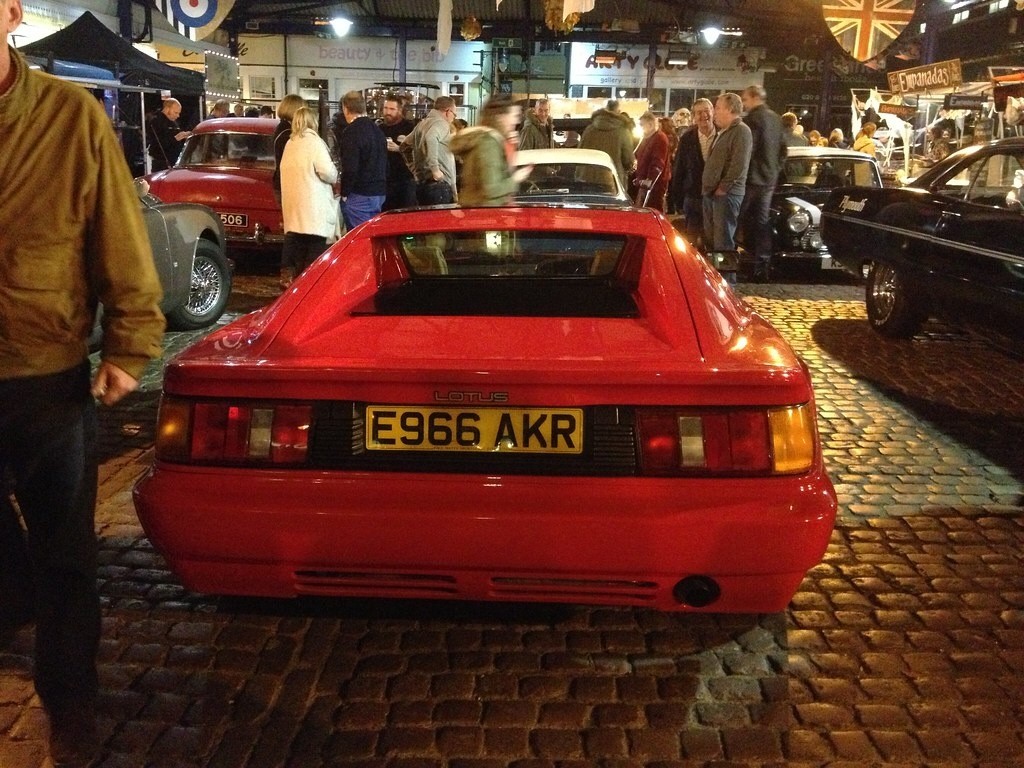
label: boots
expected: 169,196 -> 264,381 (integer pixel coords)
279,267 -> 294,290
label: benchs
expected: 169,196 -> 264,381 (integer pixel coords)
373,276 -> 643,318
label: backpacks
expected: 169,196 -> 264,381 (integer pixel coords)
832,142 -> 851,170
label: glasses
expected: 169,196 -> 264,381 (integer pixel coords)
450,110 -> 457,120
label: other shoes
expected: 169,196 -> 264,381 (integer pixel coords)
48,699 -> 102,765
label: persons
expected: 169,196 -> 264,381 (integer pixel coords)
97,83 -> 363,180
732,86 -> 789,284
0,0 -> 167,768
375,82 -> 983,248
271,94 -> 314,289
338,91 -> 392,233
279,107 -> 341,278
700,92 -> 755,291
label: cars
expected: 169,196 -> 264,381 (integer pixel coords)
142,116 -> 287,261
769,146 -> 887,272
482,147 -> 634,276
820,135 -> 1024,336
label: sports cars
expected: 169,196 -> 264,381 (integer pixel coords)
86,175 -> 236,353
128,201 -> 838,618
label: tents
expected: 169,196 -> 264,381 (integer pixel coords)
10,10 -> 206,179
